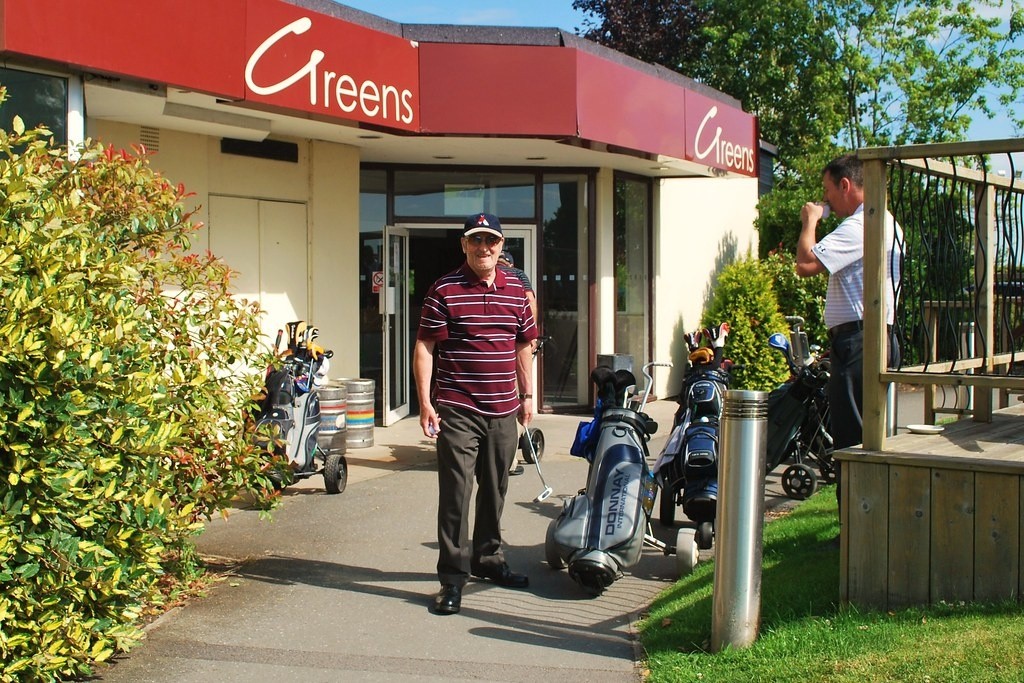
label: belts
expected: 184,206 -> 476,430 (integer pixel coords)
828,320 -> 892,338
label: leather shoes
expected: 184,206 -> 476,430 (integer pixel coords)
508,464 -> 526,475
434,584 -> 463,613
470,560 -> 529,588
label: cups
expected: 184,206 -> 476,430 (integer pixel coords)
815,202 -> 830,218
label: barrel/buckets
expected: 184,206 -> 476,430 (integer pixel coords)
316,378 -> 375,455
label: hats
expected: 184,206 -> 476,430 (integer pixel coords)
463,211 -> 505,239
495,251 -> 514,268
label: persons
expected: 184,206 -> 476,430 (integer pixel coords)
797,154 -> 906,524
413,213 -> 540,613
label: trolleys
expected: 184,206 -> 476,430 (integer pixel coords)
546,361 -> 701,594
652,322 -> 748,575
243,322 -> 348,494
763,316 -> 838,501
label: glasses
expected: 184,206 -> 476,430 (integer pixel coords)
466,235 -> 502,246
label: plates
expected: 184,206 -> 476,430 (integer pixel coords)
906,425 -> 945,434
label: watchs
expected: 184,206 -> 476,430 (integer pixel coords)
518,392 -> 532,400
